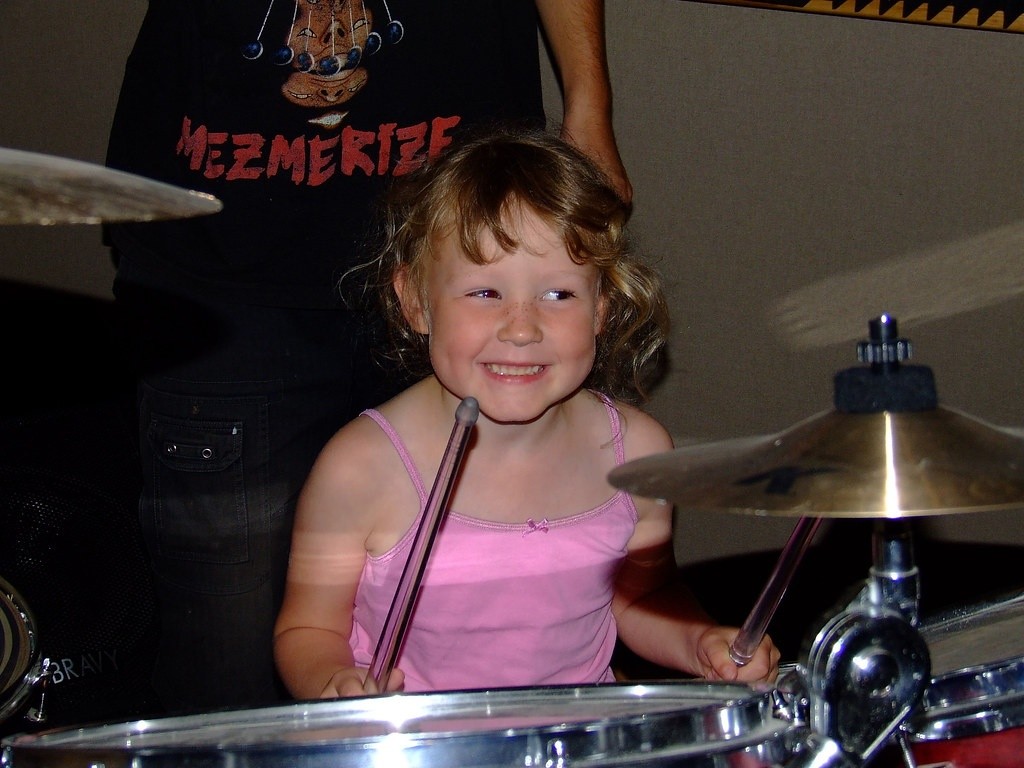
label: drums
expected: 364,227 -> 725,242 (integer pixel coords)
795,585 -> 1023,768
4,675 -> 782,768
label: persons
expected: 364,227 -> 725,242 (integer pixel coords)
274,131 -> 782,702
100,2 -> 631,723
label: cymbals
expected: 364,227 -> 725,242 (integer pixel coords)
603,298 -> 1023,529
0,142 -> 224,234
